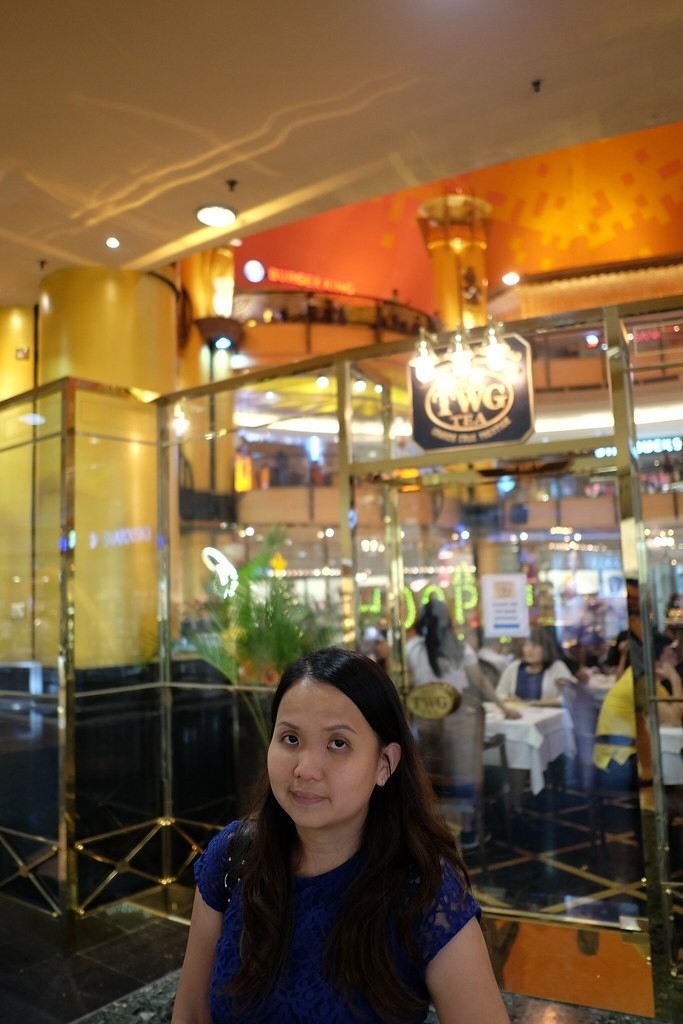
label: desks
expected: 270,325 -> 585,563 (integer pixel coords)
483,674 -> 683,834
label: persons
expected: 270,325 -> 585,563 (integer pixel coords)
592,622 -> 683,791
546,626 -> 640,678
406,596 -> 520,739
496,627 -> 579,708
172,646 -> 511,1024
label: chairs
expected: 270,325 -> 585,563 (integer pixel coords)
556,678 -> 645,875
411,694 -> 515,885
480,913 -> 655,1018
